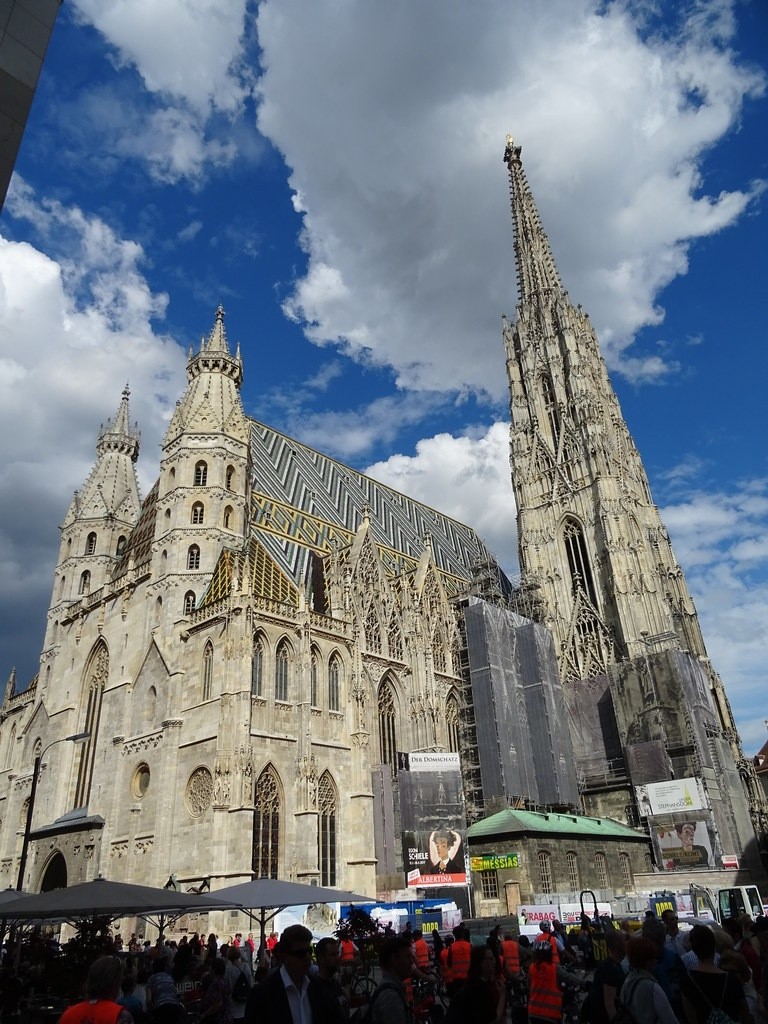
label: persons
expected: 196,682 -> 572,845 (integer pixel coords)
0,911 -> 768,1024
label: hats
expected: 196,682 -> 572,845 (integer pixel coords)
146,972 -> 175,987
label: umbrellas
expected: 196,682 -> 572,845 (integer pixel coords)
0,872 -> 380,976
1,886 -> 37,954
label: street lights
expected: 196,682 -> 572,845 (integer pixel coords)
4,733 -> 92,974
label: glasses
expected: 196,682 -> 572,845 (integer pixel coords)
484,956 -> 496,961
668,916 -> 679,921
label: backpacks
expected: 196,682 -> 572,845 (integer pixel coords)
360,983 -> 409,1024
232,962 -> 251,1003
612,977 -> 645,1024
686,971 -> 734,1024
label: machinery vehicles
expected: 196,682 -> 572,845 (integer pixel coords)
688,882 -> 766,925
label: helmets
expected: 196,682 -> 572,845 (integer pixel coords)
535,940 -> 552,952
539,920 -> 551,931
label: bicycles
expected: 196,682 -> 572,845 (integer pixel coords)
509,969 -> 528,1008
346,975 -> 378,1004
410,967 -> 450,1024
